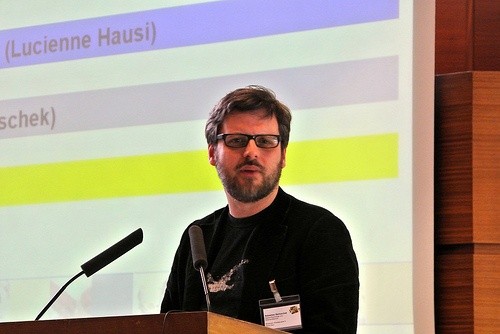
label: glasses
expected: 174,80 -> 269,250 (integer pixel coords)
216,132 -> 282,149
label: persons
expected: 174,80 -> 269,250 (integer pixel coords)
159,84 -> 362,334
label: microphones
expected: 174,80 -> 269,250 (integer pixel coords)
35,228 -> 143,321
188,225 -> 212,312
